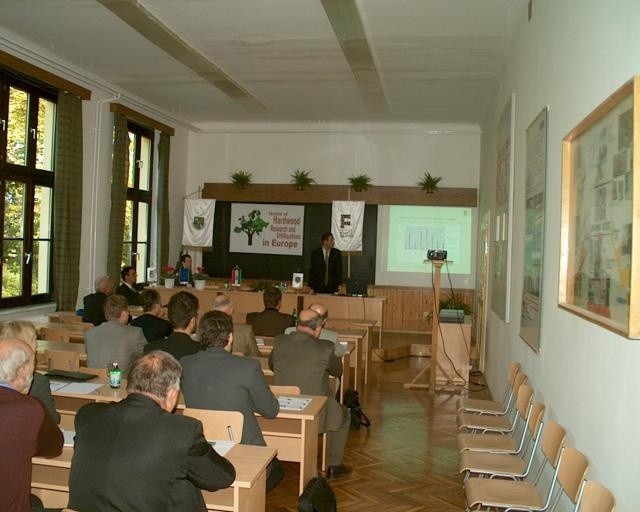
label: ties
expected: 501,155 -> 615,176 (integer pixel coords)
324,251 -> 329,286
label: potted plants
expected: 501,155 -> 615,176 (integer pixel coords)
345,174 -> 372,192
289,167 -> 317,191
416,169 -> 442,194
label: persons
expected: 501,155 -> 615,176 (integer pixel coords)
82,254 -> 205,380
212,294 -> 262,357
176,311 -> 286,493
247,285 -> 295,336
0,337 -> 64,512
283,303 -> 341,394
1,319 -> 62,425
64,349 -> 237,512
268,309 -> 352,479
308,232 -> 343,295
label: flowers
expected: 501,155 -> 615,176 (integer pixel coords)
192,267 -> 209,280
159,266 -> 176,279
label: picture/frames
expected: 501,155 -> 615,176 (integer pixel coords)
488,92 -> 517,323
557,75 -> 640,339
520,107 -> 550,354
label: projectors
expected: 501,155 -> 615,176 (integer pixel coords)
427,249 -> 448,260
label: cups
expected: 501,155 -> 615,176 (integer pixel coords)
224,282 -> 229,289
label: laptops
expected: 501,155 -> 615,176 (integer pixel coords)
345,276 -> 369,297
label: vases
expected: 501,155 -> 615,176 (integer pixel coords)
193,280 -> 206,290
164,277 -> 175,289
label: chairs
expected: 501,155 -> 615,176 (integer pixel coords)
457,361 -> 615,512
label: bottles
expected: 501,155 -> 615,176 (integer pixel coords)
110,363 -> 121,388
292,309 -> 296,322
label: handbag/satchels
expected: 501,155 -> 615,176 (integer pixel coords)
297,476 -> 336,511
344,388 -> 371,430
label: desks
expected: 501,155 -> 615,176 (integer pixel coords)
436,314 -> 472,389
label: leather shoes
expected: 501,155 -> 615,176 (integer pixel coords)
327,463 -> 351,480
